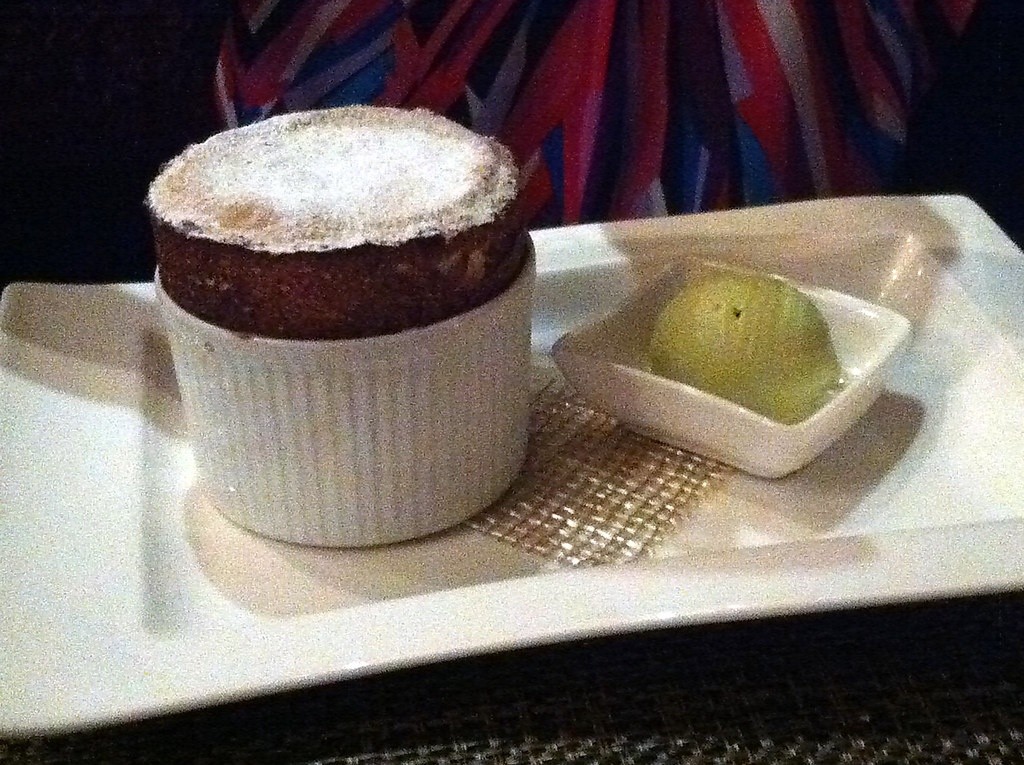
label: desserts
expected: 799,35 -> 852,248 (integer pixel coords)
141,103 -> 522,347
646,268 -> 839,428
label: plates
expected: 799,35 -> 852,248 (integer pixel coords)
551,258 -> 910,478
0,230 -> 1024,737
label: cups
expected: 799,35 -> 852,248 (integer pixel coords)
155,238 -> 536,548
